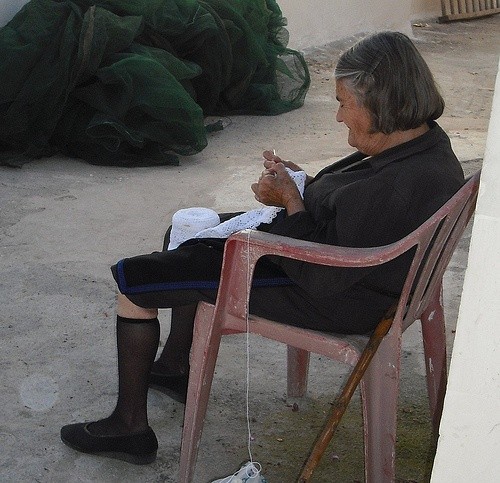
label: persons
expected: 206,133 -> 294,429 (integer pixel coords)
59,29 -> 465,466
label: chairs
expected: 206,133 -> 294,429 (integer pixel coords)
178,168 -> 483,483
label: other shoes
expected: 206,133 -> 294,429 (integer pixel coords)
60,422 -> 158,466
144,361 -> 190,404
